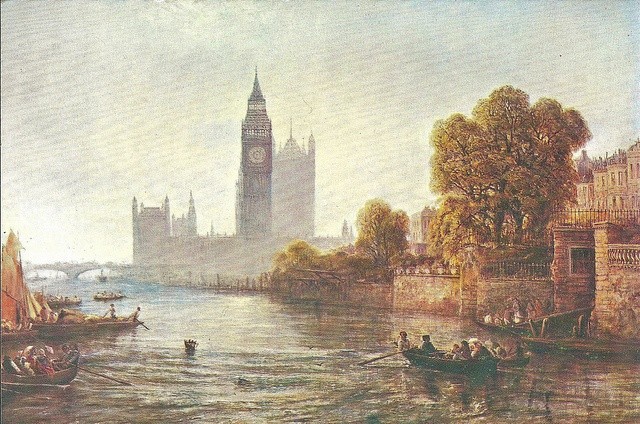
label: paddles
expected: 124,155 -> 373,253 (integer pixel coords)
354,345 -> 418,366
0,387 -> 51,400
71,363 -> 134,386
0,380 -> 71,389
135,317 -> 150,330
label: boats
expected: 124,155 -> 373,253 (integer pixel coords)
504,305 -> 595,340
32,320 -> 144,339
520,337 -> 640,363
94,295 -> 125,300
47,299 -> 82,307
473,319 -> 523,337
1,354 -> 80,385
402,349 -> 530,380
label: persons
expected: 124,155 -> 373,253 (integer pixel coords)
102,303 -> 117,317
421,334 -> 438,356
398,331 -> 422,354
446,337 -> 525,360
129,307 -> 141,321
484,299 -> 524,324
526,299 -> 543,319
34,305 -> 64,323
60,344 -> 79,369
2,344 -> 55,376
1,318 -> 33,332
97,291 -> 122,298
48,293 -> 78,301
